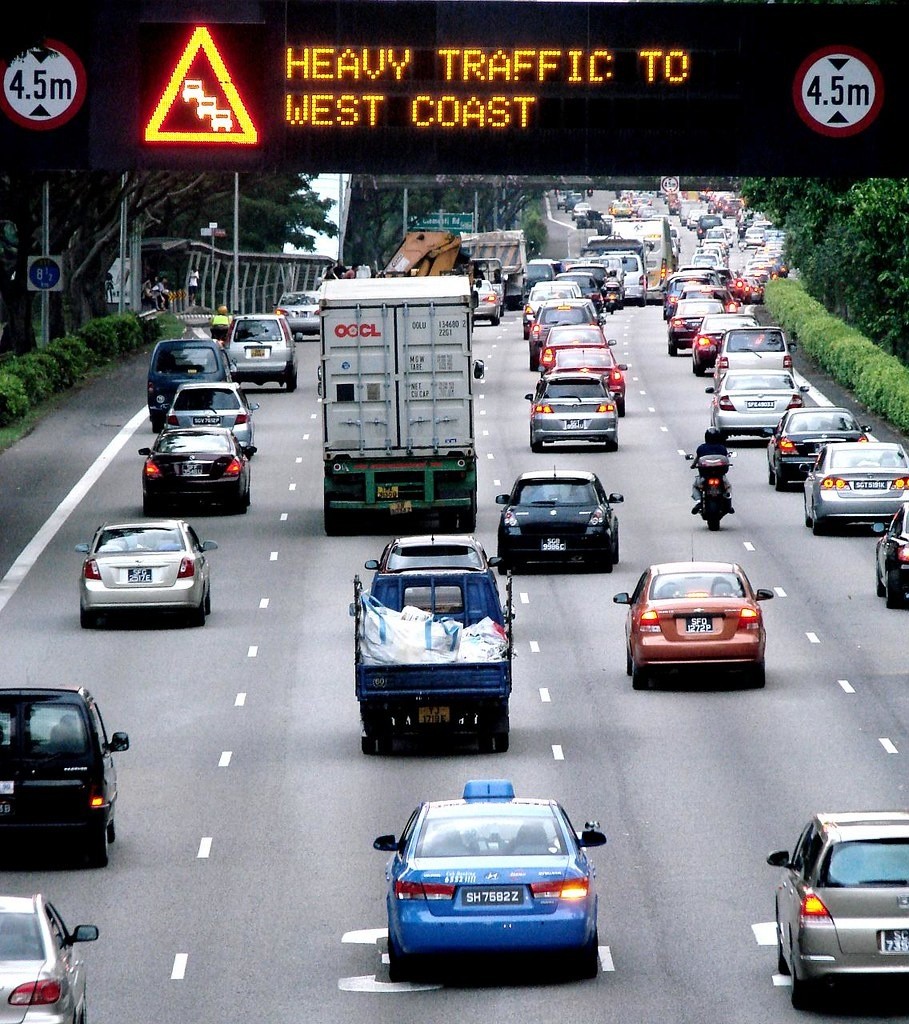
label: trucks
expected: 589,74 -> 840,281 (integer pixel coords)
319,279 -> 485,532
351,564 -> 515,761
375,228 -> 528,334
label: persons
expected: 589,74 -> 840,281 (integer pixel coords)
189,264 -> 199,304
505,823 -> 557,855
568,486 -> 593,506
491,269 -> 501,284
45,715 -> 84,752
690,426 -> 735,515
587,187 -> 593,196
324,259 -> 358,280
0,725 -> 4,745
711,577 -> 733,595
601,270 -> 623,307
141,275 -> 170,311
522,485 -> 545,503
473,264 -> 485,280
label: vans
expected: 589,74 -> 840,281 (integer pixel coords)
1,686 -> 130,873
147,339 -> 238,427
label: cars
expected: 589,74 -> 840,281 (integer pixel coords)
764,405 -> 871,491
614,562 -> 773,689
471,189 -> 810,450
495,468 -> 623,573
138,381 -> 259,515
74,521 -> 217,626
0,895 -> 99,1022
374,781 -> 608,982
272,291 -> 320,341
873,502 -> 909,612
799,441 -> 909,537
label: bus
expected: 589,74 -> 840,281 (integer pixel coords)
609,219 -> 674,304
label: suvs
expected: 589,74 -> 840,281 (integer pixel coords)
224,314 -> 303,391
766,811 -> 909,1011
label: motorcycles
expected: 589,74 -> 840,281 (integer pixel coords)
685,452 -> 738,532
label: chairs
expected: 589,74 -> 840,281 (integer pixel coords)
714,583 -> 730,595
661,585 -> 681,597
106,540 -> 125,548
430,844 -> 469,856
158,539 -> 174,548
515,844 -> 551,855
881,458 -> 895,467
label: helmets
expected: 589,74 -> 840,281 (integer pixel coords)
217,306 -> 228,315
705,427 -> 721,445
609,270 -> 617,277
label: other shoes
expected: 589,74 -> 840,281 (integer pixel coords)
730,508 -> 734,514
691,502 -> 702,514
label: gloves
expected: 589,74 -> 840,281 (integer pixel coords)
691,464 -> 696,469
